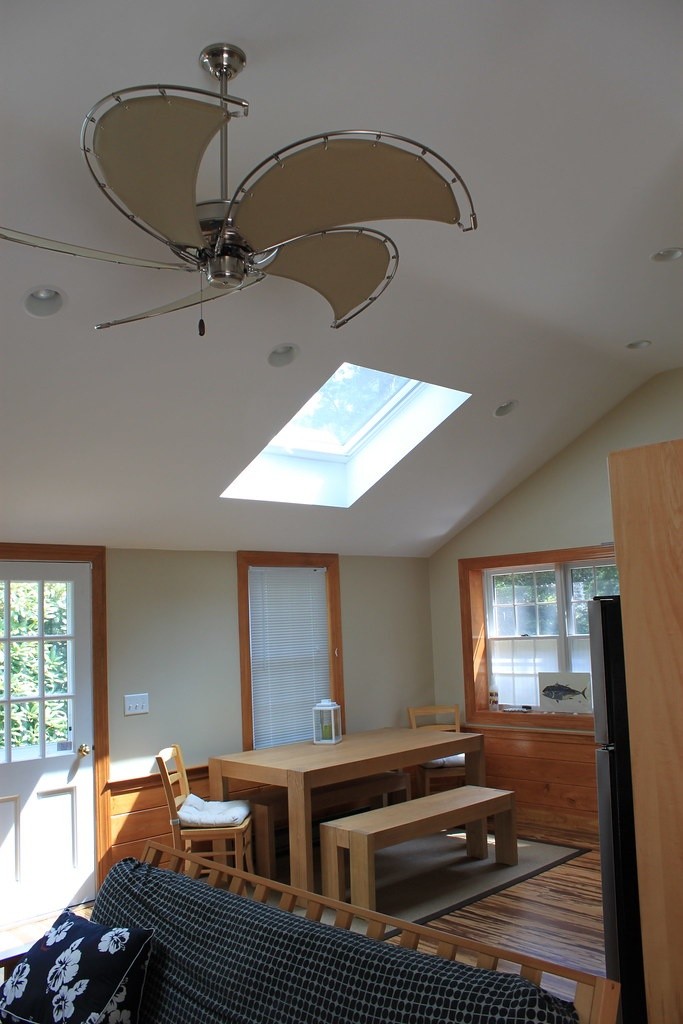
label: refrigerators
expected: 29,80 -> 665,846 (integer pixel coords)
586,595 -> 649,1024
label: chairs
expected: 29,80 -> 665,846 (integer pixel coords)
155,744 -> 256,898
407,704 -> 467,798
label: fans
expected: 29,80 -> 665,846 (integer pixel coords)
0,42 -> 477,335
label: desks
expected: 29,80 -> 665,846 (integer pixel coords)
208,726 -> 489,908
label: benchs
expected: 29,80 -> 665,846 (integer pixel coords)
319,785 -> 518,922
232,770 -> 414,894
0,842 -> 620,1024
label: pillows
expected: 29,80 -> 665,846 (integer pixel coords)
0,907 -> 156,1023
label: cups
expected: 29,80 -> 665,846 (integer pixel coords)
487,685 -> 500,711
